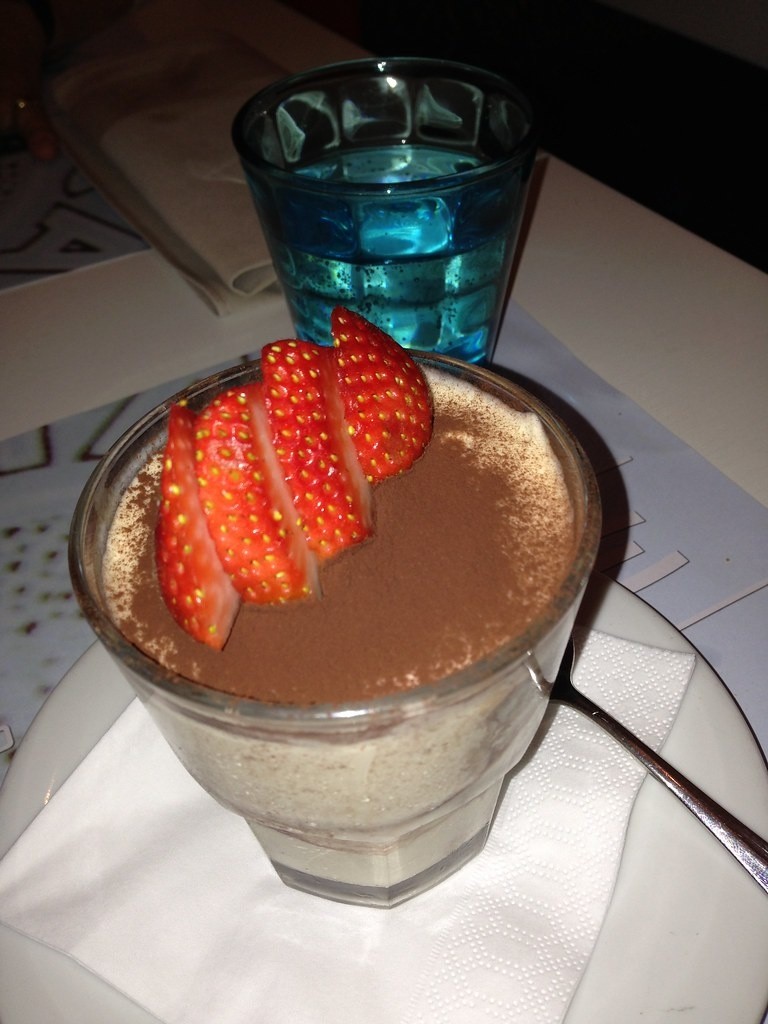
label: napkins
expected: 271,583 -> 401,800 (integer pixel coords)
0,631 -> 698,1024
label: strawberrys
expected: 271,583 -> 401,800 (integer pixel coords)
156,307 -> 433,651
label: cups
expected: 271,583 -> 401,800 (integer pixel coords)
68,347 -> 603,907
229,54 -> 534,365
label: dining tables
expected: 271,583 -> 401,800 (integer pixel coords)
0,0 -> 768,1024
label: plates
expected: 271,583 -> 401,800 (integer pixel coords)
0,575 -> 767,1024
